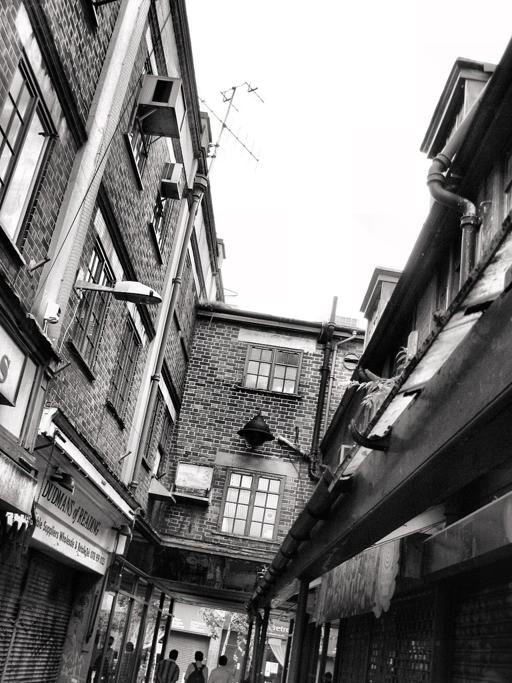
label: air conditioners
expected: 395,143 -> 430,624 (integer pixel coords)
160,161 -> 186,200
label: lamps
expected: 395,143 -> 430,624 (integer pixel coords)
73,279 -> 163,306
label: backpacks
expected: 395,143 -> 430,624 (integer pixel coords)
187,663 -> 206,683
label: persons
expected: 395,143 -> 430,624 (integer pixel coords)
154,649 -> 179,682
97,636 -> 115,683
208,654 -> 235,682
184,650 -> 208,683
120,642 -> 141,681
